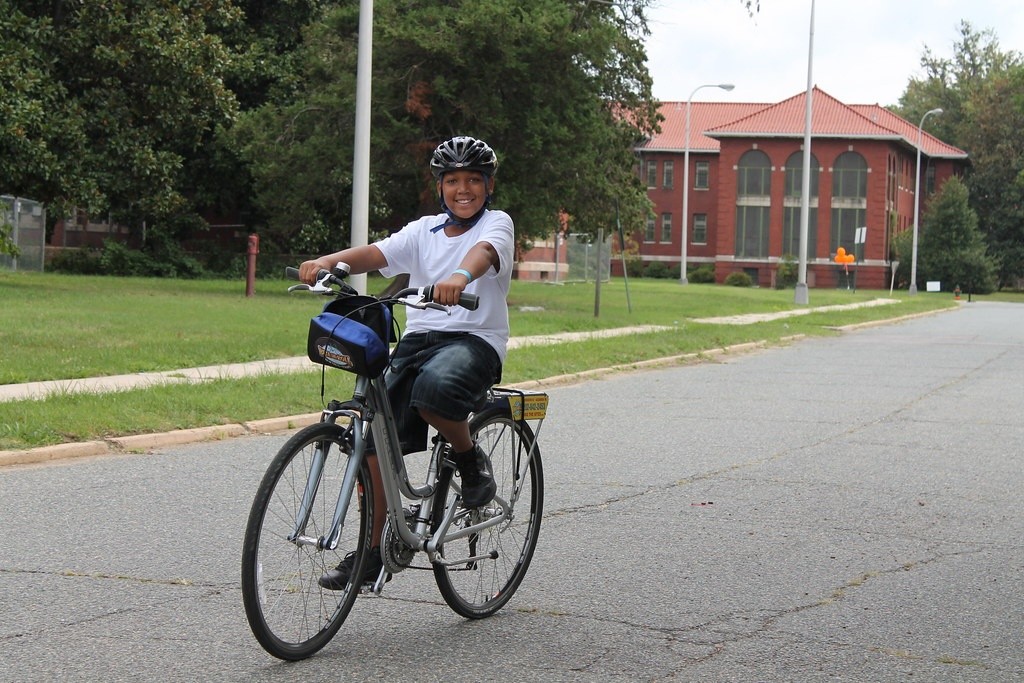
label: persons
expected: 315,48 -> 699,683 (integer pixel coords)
299,137 -> 514,590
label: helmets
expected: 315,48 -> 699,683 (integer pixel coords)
429,134 -> 499,177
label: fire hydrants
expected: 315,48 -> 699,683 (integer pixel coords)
953,284 -> 962,300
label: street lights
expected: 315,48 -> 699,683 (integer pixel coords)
676,83 -> 739,286
906,108 -> 944,295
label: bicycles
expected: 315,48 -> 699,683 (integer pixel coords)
241,264 -> 546,663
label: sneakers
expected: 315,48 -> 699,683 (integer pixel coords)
318,547 -> 392,589
448,440 -> 496,508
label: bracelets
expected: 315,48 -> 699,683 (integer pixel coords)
450,268 -> 472,283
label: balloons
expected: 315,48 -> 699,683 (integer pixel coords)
833,246 -> 856,263
